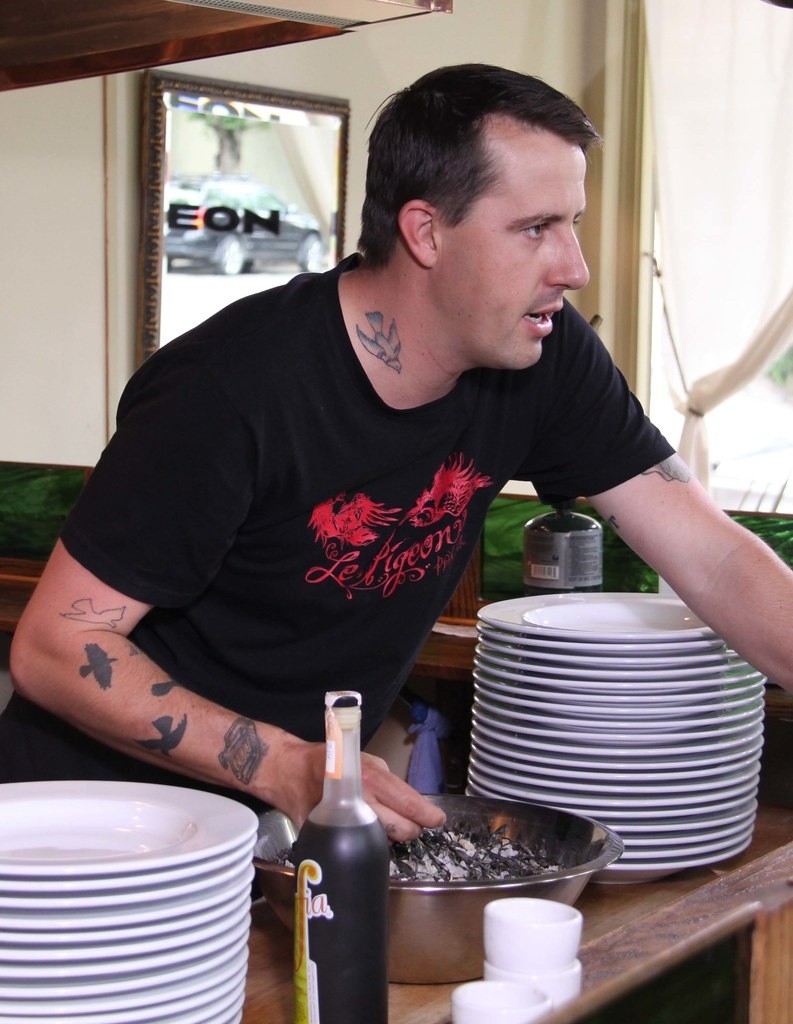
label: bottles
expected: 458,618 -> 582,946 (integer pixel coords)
291,688 -> 391,1024
525,499 -> 602,597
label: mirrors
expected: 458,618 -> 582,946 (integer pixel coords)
138,66 -> 351,367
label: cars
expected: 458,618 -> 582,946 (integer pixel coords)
163,178 -> 326,274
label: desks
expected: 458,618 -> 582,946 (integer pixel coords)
244,793 -> 792,1022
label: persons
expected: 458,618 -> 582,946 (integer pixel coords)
0,60 -> 792,831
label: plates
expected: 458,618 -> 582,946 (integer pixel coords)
0,780 -> 258,1024
465,592 -> 764,884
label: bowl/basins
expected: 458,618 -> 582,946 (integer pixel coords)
483,957 -> 582,1011
484,897 -> 583,965
450,981 -> 553,1024
248,793 -> 624,984
